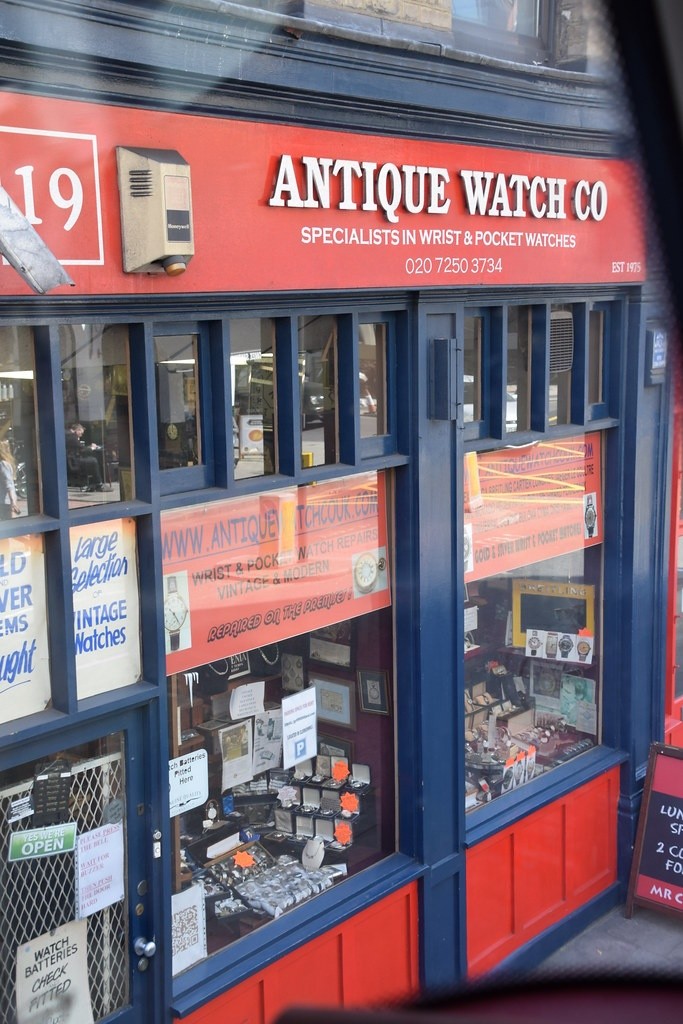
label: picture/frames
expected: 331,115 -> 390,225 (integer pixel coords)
307,671 -> 357,731
281,650 -> 306,693
357,668 -> 392,716
306,618 -> 356,672
316,730 -> 354,775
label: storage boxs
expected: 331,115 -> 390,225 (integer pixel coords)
464,563 -> 600,815
173,756 -> 373,936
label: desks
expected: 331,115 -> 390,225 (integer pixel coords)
86,443 -> 117,488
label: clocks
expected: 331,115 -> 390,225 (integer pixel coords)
246,360 -> 305,429
166,423 -> 179,440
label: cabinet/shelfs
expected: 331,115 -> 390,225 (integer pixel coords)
0,399 -> 14,455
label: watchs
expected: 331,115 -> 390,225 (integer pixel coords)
528,631 -> 591,662
465,691 -> 591,764
164,577 -> 189,651
293,770 -> 363,788
503,757 -> 534,790
354,553 -> 386,593
464,638 -> 470,648
464,526 -> 470,572
585,494 -> 596,538
281,800 -> 352,818
273,832 -> 343,849
198,845 -> 331,917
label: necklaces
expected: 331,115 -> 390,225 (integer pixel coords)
259,643 -> 279,665
208,658 -> 229,675
306,842 -> 322,859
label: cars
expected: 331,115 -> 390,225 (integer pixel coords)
236,364 -> 328,427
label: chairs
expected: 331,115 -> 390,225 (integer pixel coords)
67,447 -> 93,490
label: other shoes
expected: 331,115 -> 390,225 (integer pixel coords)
98,482 -> 115,492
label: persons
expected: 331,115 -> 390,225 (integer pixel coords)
65,424 -> 114,492
0,441 -> 21,521
